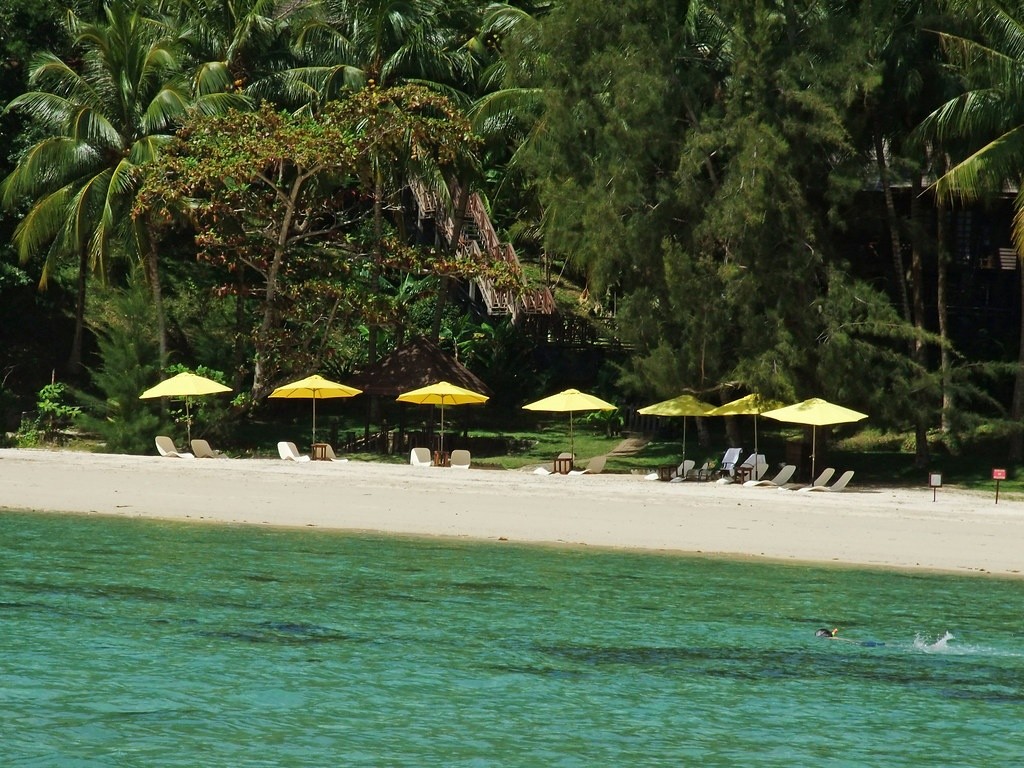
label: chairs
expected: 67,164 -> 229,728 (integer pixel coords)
155,436 -> 194,459
450,449 -> 471,469
410,447 -> 434,467
644,460 -> 695,481
278,442 -> 310,462
532,453 -> 575,475
311,443 -> 348,462
670,448 -> 855,493
567,456 -> 606,475
191,439 -> 229,458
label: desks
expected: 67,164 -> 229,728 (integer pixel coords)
434,451 -> 450,467
554,458 -> 572,475
311,445 -> 327,461
657,464 -> 753,485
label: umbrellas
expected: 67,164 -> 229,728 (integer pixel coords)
759,398 -> 868,487
268,374 -> 363,460
636,394 -> 717,478
522,389 -> 619,471
395,381 -> 490,468
138,370 -> 234,453
703,392 -> 790,484
